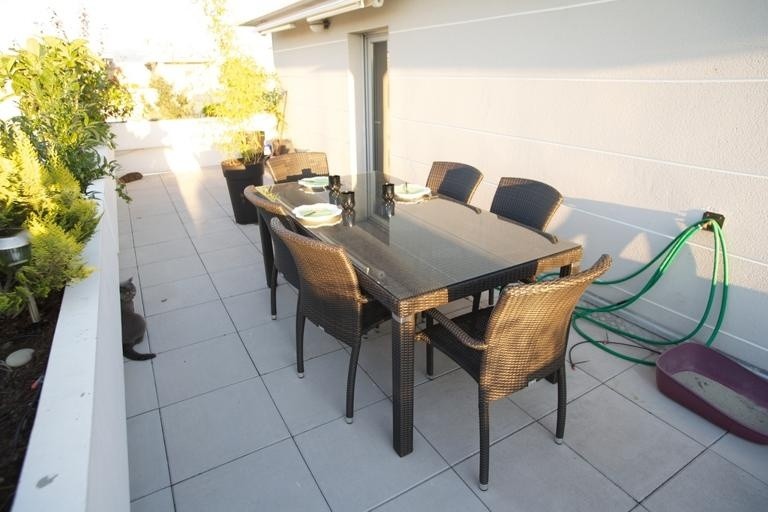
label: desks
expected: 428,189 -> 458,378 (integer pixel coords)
253,169 -> 587,457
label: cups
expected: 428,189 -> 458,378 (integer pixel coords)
329,190 -> 341,206
340,191 -> 355,210
382,183 -> 394,202
328,175 -> 340,190
341,209 -> 356,227
384,201 -> 395,216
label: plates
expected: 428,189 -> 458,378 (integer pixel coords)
392,183 -> 431,199
296,176 -> 338,188
291,202 -> 343,222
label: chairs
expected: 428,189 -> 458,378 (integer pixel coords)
265,151 -> 331,182
487,174 -> 565,309
423,160 -> 485,205
269,217 -> 390,426
243,183 -> 301,322
417,254 -> 612,492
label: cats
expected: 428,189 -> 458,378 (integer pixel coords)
119,276 -> 156,361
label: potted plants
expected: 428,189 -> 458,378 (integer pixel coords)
192,0 -> 287,225
